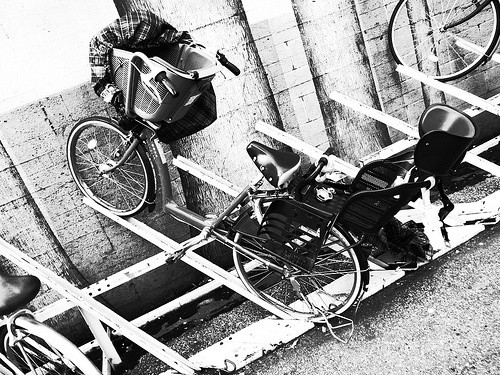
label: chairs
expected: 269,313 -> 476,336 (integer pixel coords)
257,103 -> 480,273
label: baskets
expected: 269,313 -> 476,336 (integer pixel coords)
108,38 -> 223,124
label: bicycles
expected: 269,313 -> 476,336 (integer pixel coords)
388,0 -> 500,81
66,24 -> 479,344
0,276 -> 100,372
